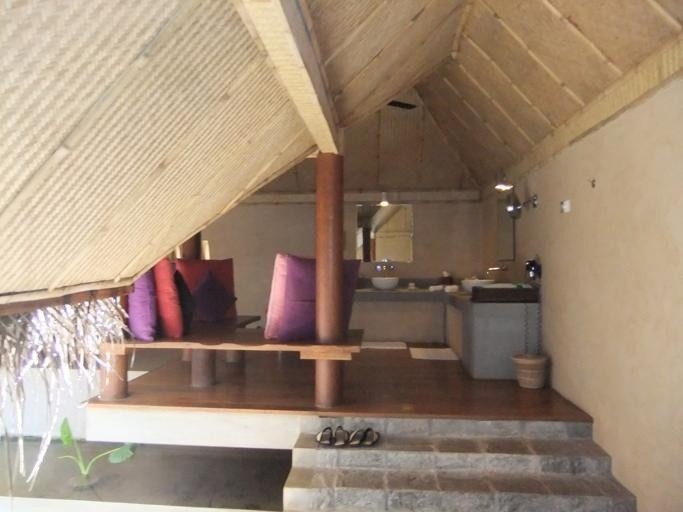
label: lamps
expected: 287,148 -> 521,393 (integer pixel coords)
499,177 -> 537,219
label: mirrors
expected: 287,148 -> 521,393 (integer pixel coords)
354,204 -> 413,263
496,198 -> 515,261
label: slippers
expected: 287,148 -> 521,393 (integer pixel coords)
363,427 -> 379,445
315,427 -> 332,445
335,425 -> 348,446
350,428 -> 363,446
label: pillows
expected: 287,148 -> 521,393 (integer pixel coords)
263,252 -> 361,341
152,256 -> 184,340
177,256 -> 237,320
127,271 -> 158,340
191,269 -> 238,325
174,270 -> 194,335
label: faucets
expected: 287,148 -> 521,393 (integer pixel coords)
486,264 -> 508,275
376,264 -> 396,272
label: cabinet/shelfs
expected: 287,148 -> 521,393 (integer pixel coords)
349,288 -> 445,348
446,287 -> 541,381
